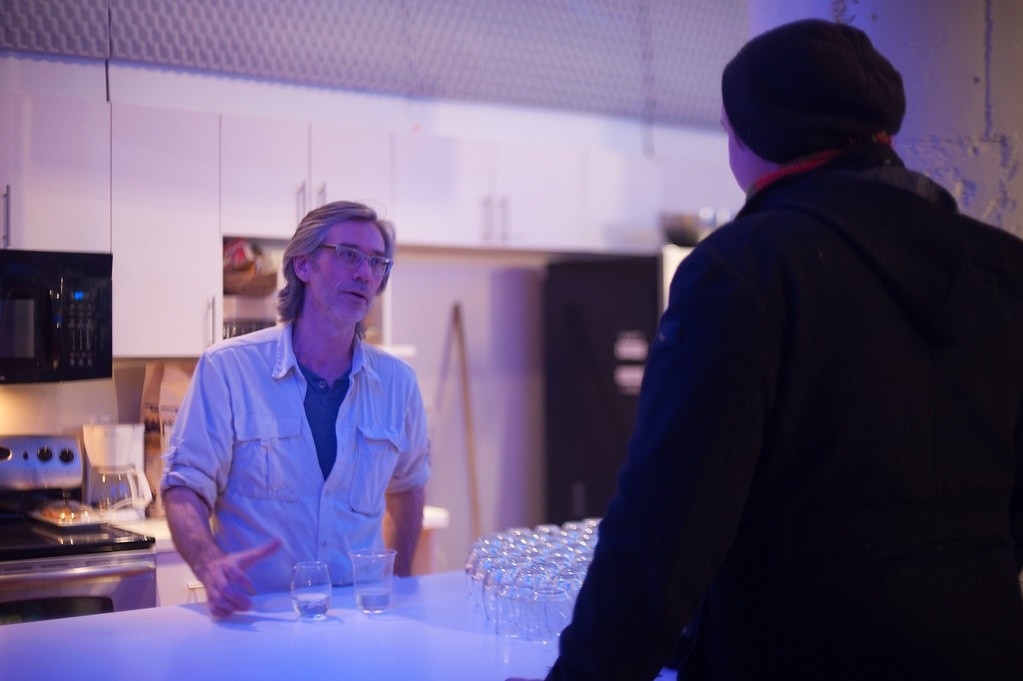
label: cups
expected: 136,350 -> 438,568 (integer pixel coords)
347,548 -> 399,614
290,561 -> 333,621
464,517 -> 603,642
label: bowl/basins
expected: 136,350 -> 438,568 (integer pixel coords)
653,209 -> 737,247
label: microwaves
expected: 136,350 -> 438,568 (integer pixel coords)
0,248 -> 114,385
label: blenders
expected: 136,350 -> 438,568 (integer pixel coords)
82,423 -> 153,522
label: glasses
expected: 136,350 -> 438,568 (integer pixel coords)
317,243 -> 393,276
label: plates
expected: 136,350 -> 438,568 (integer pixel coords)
28,511 -> 107,532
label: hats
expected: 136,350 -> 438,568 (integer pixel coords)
721,20 -> 906,165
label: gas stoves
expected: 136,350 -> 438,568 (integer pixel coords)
0,434 -> 156,561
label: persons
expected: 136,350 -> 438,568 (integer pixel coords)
159,200 -> 430,617
544,18 -> 1023,681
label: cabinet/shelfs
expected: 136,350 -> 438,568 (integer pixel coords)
0,90 -> 749,361
156,530 -> 437,606
